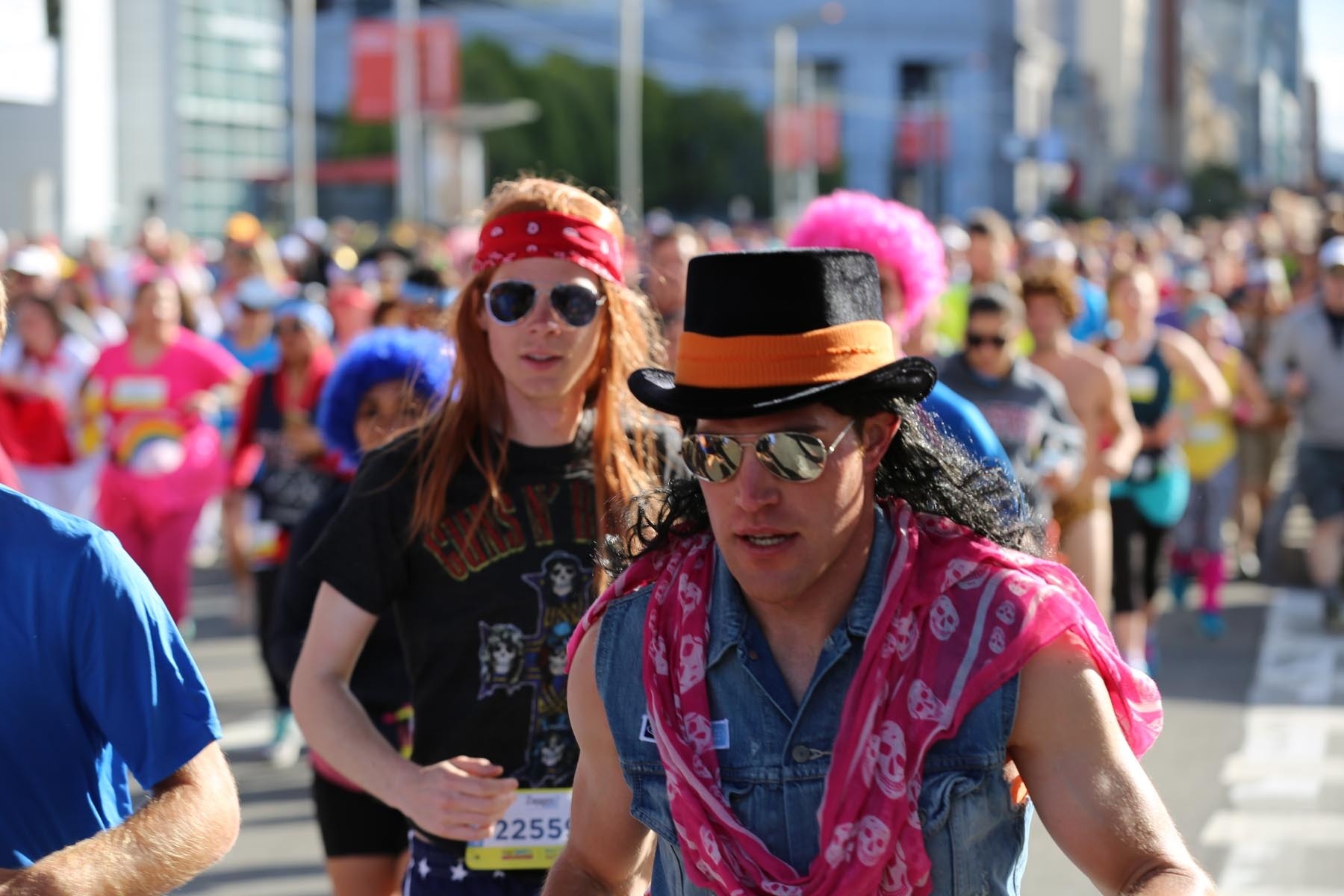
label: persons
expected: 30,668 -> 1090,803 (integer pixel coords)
0,162 -> 1344,768
0,281 -> 241,896
541,249 -> 1218,896
288,176 -> 692,896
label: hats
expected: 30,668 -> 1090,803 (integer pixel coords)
627,247 -> 937,422
2,245 -> 59,286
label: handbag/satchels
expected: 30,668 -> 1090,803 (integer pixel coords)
1134,455 -> 1189,526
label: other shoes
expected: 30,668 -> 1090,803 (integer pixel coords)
1168,569 -> 1192,597
267,713 -> 305,768
1325,587 -> 1343,631
1197,609 -> 1223,639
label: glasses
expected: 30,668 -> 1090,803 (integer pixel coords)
271,317 -> 311,334
967,333 -> 1008,350
677,418 -> 859,484
481,280 -> 608,328
